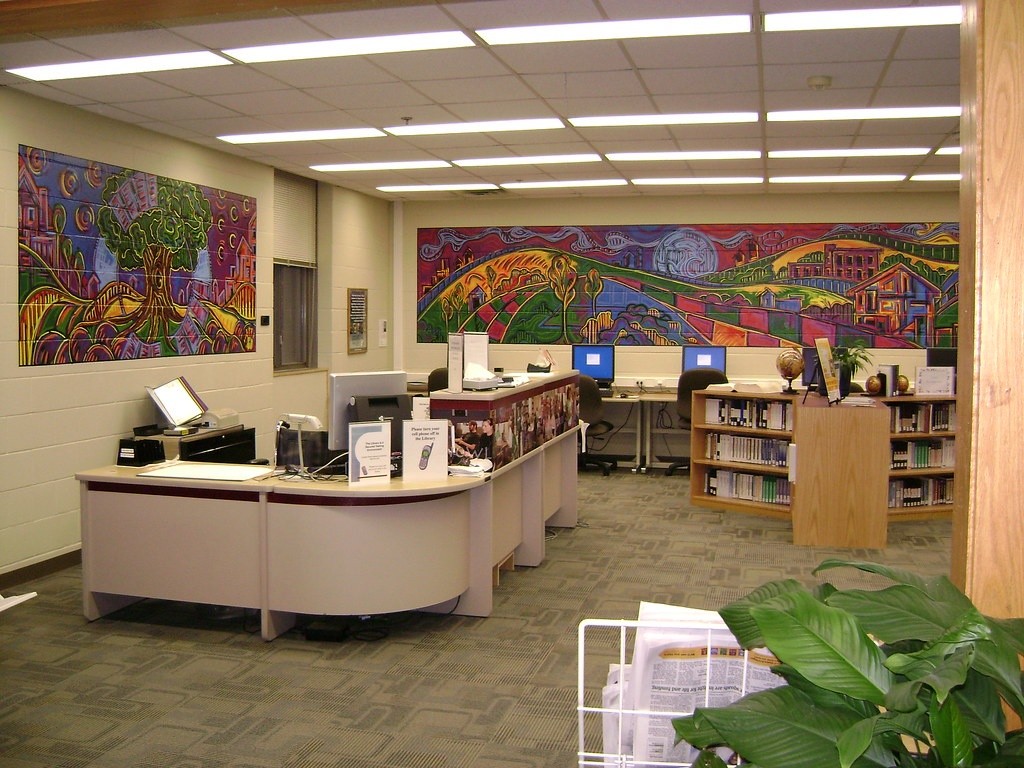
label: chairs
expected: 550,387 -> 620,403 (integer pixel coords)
579,375 -> 617,475
663,370 -> 728,476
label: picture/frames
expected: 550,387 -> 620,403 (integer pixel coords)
347,288 -> 368,356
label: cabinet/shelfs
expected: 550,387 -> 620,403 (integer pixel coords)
690,389 -> 956,551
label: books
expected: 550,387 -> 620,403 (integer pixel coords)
887,405 -> 957,508
841,397 -> 875,404
703,398 -> 793,505
879,365 -> 899,397
704,381 -> 782,393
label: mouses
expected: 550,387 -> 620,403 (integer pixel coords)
620,393 -> 626,398
248,458 -> 269,465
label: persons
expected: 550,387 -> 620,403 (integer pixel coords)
567,387 -> 574,427
450,418 -> 495,472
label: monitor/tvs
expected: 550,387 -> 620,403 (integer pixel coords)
927,347 -> 958,394
572,344 -> 615,388
803,347 -> 848,391
682,345 -> 726,376
327,371 -> 408,450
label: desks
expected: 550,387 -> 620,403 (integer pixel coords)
76,387 -> 689,641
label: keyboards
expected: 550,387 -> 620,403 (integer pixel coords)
307,465 -> 346,475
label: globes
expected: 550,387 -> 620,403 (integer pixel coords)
896,374 -> 915,397
865,375 -> 881,396
775,346 -> 805,395
